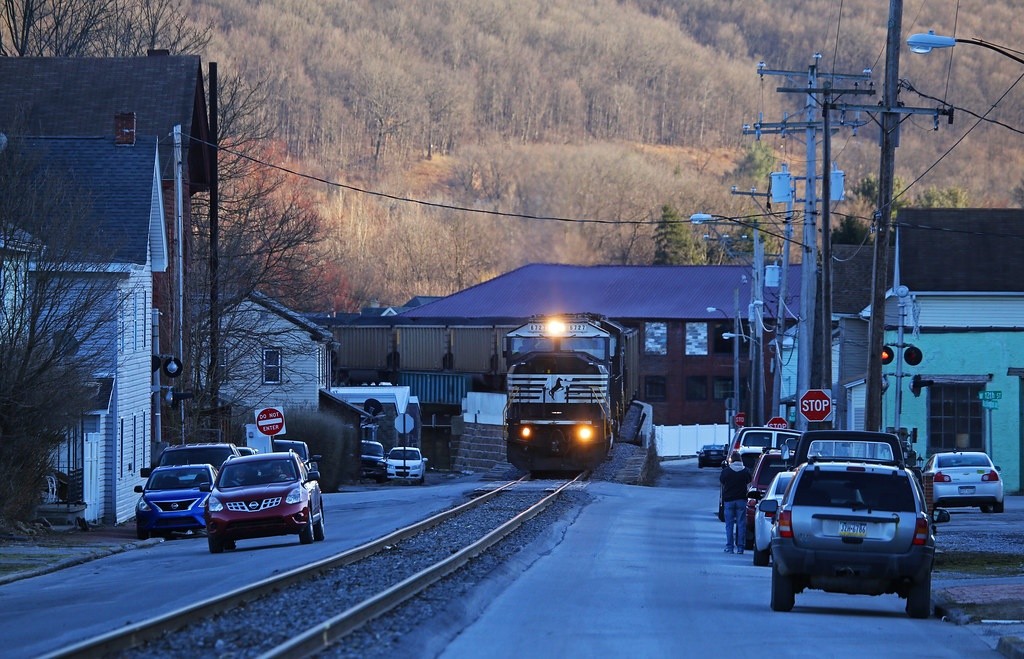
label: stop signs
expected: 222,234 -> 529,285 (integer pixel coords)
734,412 -> 746,426
800,389 -> 832,422
767,417 -> 788,428
255,405 -> 287,437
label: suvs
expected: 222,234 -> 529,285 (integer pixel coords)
759,456 -> 951,618
781,429 -> 905,467
719,426 -> 803,523
140,444 -> 243,483
747,447 -> 794,552
199,448 -> 325,552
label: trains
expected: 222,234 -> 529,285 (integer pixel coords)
501,312 -> 641,471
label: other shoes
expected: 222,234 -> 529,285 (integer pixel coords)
737,551 -> 743,554
724,547 -> 734,554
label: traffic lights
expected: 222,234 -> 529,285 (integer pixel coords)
904,346 -> 922,366
882,346 -> 893,365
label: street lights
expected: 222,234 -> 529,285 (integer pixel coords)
706,298 -> 784,417
691,212 -> 816,434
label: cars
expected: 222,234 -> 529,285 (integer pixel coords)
739,448 -> 763,471
696,445 -> 726,469
361,441 -> 387,475
748,470 -> 798,567
384,447 -> 428,485
235,440 -> 322,474
921,451 -> 1005,514
133,463 -> 220,538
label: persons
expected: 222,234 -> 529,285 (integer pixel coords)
272,464 -> 291,478
720,453 -> 752,554
223,466 -> 246,485
